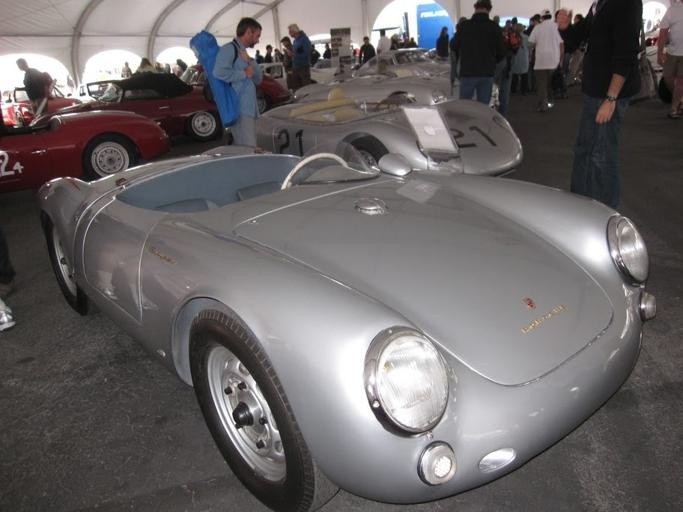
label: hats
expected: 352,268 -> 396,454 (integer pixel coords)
540,10 -> 551,17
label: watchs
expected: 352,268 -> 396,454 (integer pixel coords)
607,95 -> 619,104
656,0 -> 683,118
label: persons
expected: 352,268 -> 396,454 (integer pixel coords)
559,1 -> 644,211
0,231 -> 17,332
449,9 -> 600,110
359,29 -> 418,64
213,17 -> 262,149
121,59 -> 187,79
436,27 -> 449,60
450,0 -> 507,105
255,24 -> 332,91
16,59 -> 48,113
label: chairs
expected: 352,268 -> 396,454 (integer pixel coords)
154,198 -> 218,213
321,107 -> 361,121
237,181 -> 293,201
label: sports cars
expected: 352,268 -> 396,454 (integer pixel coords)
2,86 -> 82,127
1,106 -> 172,191
60,73 -> 220,143
36,144 -> 657,508
254,93 -> 523,177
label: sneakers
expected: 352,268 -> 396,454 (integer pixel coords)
0,307 -> 14,331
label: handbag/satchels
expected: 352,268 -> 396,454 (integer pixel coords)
629,59 -> 658,101
